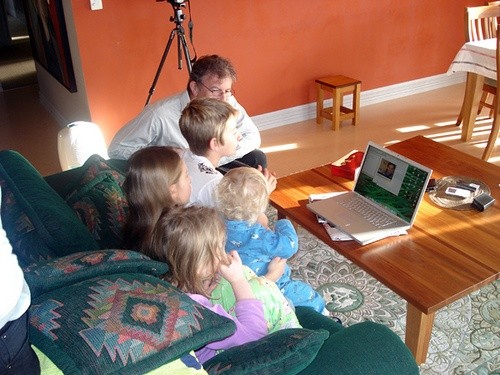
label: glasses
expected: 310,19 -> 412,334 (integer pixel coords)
198,81 -> 235,97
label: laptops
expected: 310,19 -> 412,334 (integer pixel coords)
305,141 -> 433,247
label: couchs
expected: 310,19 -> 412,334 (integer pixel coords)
0,149 -> 418,375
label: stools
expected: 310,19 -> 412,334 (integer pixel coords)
315,75 -> 362,129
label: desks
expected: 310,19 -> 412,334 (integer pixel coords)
446,38 -> 497,141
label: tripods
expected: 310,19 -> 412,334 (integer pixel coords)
142,8 -> 193,108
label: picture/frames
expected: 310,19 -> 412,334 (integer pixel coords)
22,0 -> 77,93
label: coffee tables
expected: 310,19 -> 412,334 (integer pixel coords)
268,134 -> 500,366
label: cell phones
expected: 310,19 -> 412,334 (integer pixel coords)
455,178 -> 480,192
445,187 -> 470,198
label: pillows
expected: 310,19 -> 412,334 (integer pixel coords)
23,155 -> 329,375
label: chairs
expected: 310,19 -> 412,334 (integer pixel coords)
455,1 -> 500,161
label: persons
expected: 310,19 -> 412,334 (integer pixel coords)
178,98 -> 277,209
108,53 -> 268,176
381,162 -> 395,181
141,204 -> 301,364
118,146 -> 191,253
0,187 -> 41,375
214,167 -> 329,317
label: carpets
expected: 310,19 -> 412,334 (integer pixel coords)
260,204 -> 500,375
